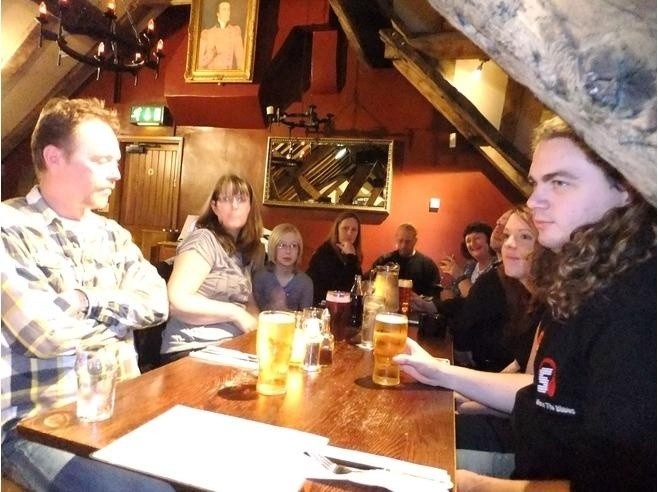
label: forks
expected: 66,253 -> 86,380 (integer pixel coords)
306,447 -> 442,486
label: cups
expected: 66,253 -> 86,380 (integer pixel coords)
360,294 -> 387,343
301,306 -> 323,321
398,279 -> 413,314
75,342 -> 120,422
255,311 -> 295,396
372,312 -> 408,385
327,290 -> 352,342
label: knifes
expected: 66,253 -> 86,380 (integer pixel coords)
304,446 -> 388,472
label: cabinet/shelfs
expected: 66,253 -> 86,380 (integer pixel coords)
157,239 -> 178,282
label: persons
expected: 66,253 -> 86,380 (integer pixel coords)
373,223 -> 441,300
412,207 -> 515,373
196,2 -> 244,73
158,173 -> 258,367
455,203 -> 538,479
252,223 -> 313,311
441,220 -> 498,297
390,114 -> 657,488
1,95 -> 176,492
304,214 -> 364,308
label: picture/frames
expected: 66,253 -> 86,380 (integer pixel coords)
262,136 -> 395,216
183,0 -> 260,86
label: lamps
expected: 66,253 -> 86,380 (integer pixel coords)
447,126 -> 458,153
32,0 -> 164,86
473,61 -> 484,80
266,104 -> 334,137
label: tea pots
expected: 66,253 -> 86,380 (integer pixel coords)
371,264 -> 400,312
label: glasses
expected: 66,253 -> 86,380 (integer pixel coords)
277,241 -> 298,250
217,194 -> 248,202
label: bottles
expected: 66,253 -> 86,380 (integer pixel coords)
318,307 -> 336,367
348,273 -> 364,328
301,318 -> 324,371
